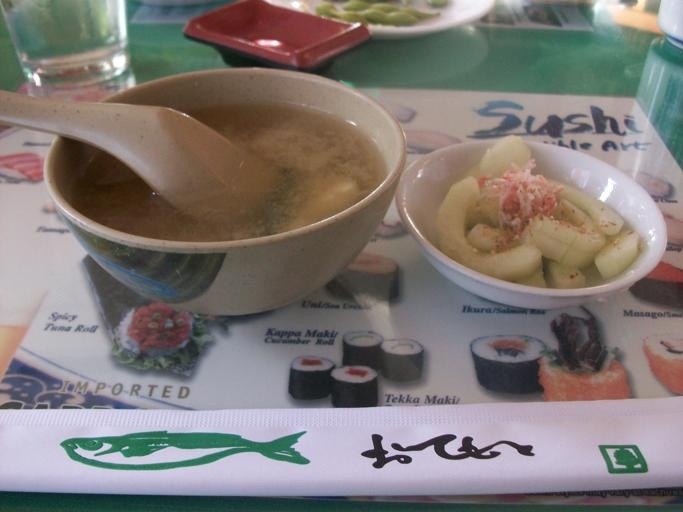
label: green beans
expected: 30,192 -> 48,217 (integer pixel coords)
319,0 -> 451,28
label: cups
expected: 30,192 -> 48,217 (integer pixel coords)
0,0 -> 129,89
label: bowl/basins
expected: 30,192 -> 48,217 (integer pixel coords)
396,136 -> 669,313
44,67 -> 408,319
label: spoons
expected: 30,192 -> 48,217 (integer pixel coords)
0,86 -> 266,222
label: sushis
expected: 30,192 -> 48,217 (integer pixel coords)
328,249 -> 400,300
663,213 -> 683,252
539,307 -> 632,400
630,257 -> 683,305
289,331 -> 423,405
642,334 -> 682,397
471,334 -> 547,391
630,171 -> 674,201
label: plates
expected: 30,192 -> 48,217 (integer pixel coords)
179,0 -> 372,75
265,0 -> 496,40
